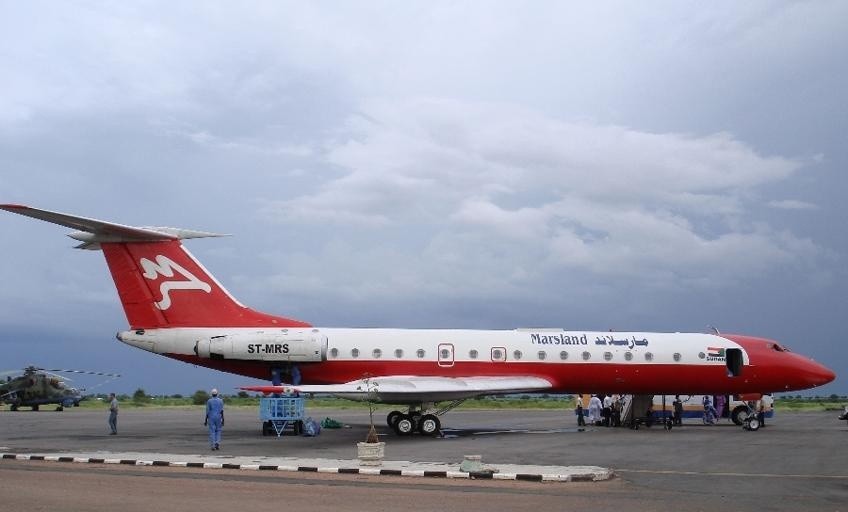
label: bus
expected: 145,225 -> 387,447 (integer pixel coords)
575,393 -> 772,426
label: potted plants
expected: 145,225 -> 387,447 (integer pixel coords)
354,372 -> 387,467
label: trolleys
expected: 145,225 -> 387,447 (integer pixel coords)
259,396 -> 303,436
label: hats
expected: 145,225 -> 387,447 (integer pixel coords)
212,389 -> 217,394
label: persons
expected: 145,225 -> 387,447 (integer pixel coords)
272,365 -> 283,397
574,393 -> 626,426
290,364 -> 300,387
109,393 -> 119,434
757,399 -> 765,428
205,389 -> 225,451
7,376 -> 17,399
672,395 -> 683,427
703,395 -> 726,425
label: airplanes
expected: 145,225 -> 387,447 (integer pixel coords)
0,202 -> 836,437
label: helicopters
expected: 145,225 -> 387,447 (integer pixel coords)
0,363 -> 121,411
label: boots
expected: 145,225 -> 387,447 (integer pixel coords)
211,443 -> 220,450
